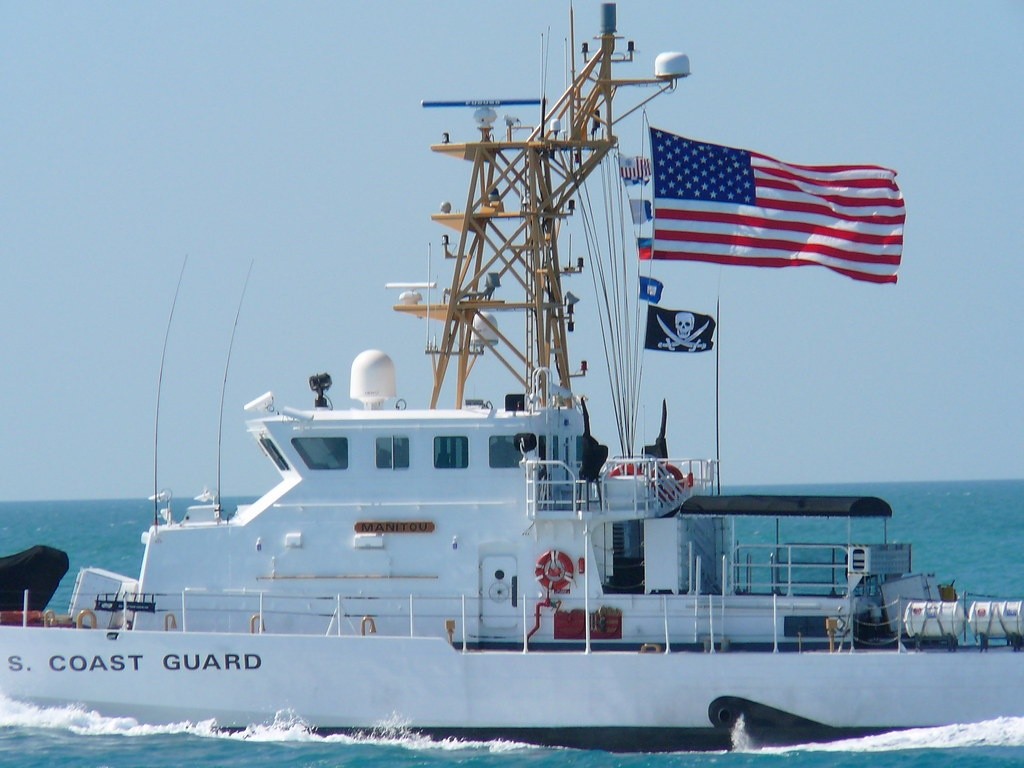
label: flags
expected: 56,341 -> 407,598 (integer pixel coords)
651,126 -> 907,285
644,304 -> 717,353
618,153 -> 663,304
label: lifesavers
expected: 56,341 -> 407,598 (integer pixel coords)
535,551 -> 574,590
650,464 -> 685,501
609,464 -> 644,476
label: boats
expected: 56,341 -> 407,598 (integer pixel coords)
0,0 -> 1024,746
